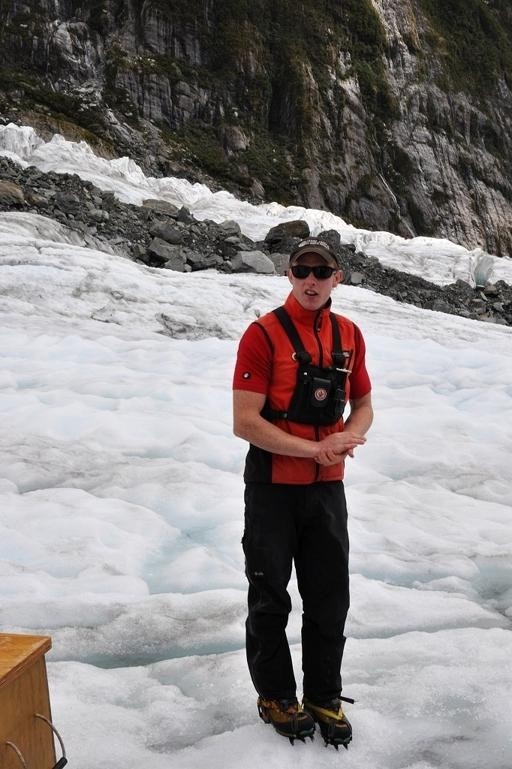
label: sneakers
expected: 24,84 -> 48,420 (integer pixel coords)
300,692 -> 354,746
254,694 -> 316,740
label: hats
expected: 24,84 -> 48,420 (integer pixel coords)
288,237 -> 339,267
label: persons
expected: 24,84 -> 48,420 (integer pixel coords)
233,239 -> 374,751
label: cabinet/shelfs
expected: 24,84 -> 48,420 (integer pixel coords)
0,629 -> 67,767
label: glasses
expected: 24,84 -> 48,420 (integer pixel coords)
287,264 -> 337,279
